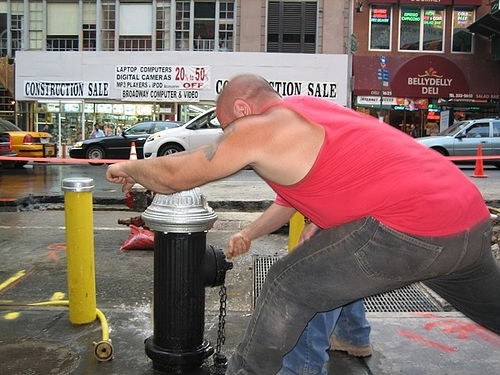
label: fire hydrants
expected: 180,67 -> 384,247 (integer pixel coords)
141,187 -> 233,371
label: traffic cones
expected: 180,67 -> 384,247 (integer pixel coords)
471,144 -> 487,178
129,141 -> 138,159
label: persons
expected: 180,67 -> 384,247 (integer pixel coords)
104,70 -> 498,374
223,190 -> 373,375
89,123 -> 105,138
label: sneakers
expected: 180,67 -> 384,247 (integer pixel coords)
329,335 -> 372,357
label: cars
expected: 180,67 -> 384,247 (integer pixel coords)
69,121 -> 185,166
0,119 -> 57,168
414,119 -> 500,169
143,106 -> 225,158
